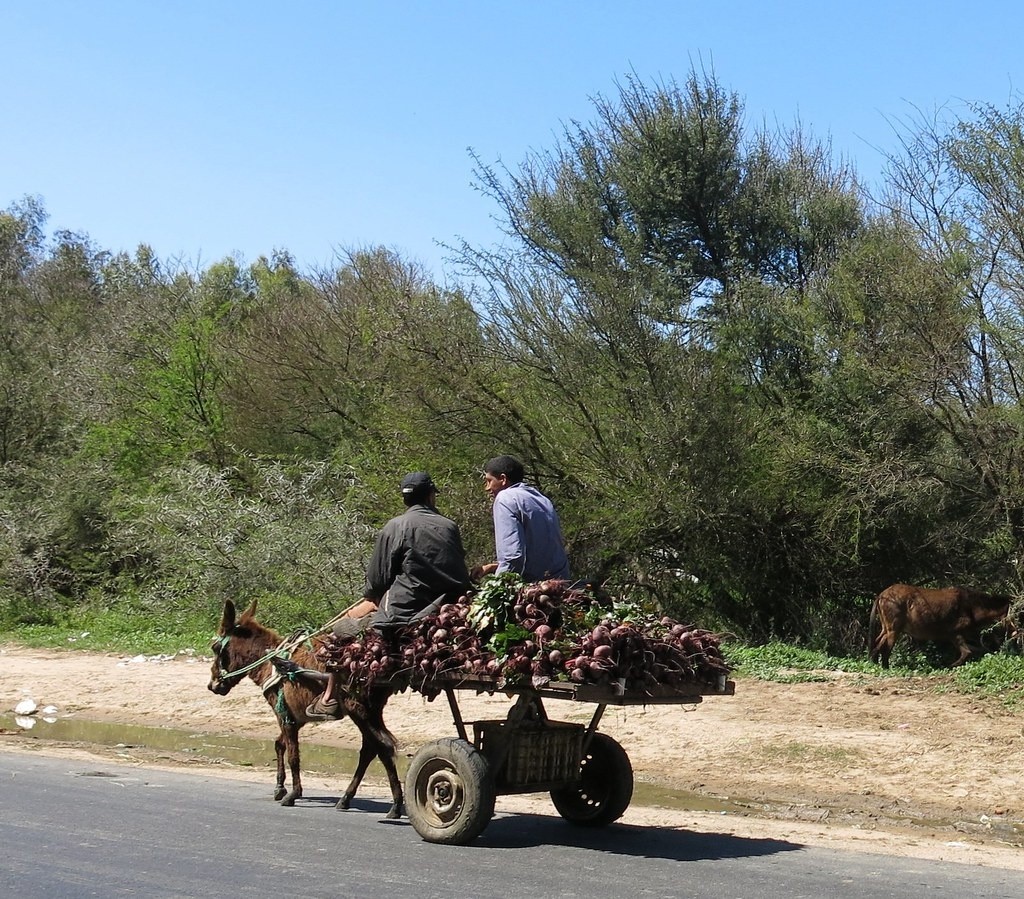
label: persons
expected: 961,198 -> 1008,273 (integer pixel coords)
361,471 -> 465,637
470,455 -> 571,583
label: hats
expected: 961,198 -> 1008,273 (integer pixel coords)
401,473 -> 441,496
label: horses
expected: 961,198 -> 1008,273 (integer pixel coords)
206,598 -> 403,824
866,583 -> 1024,670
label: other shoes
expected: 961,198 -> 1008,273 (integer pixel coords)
306,691 -> 344,720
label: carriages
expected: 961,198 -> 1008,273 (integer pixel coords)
205,572 -> 737,844
